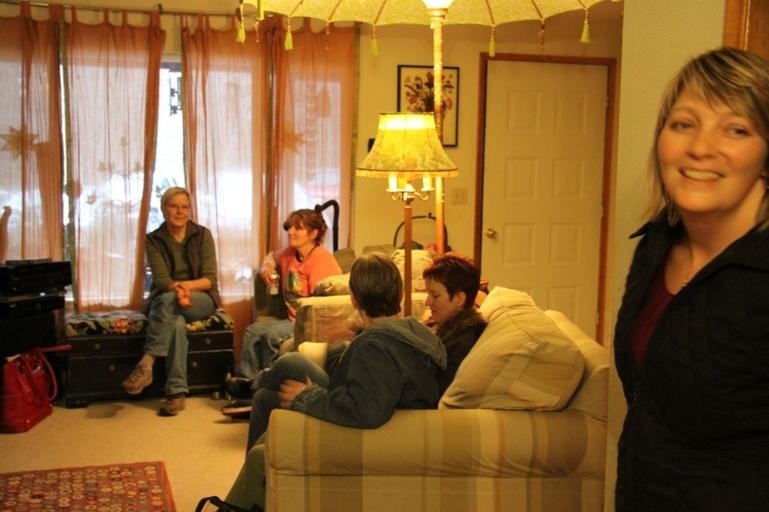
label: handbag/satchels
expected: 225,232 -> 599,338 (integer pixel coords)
1,349 -> 57,435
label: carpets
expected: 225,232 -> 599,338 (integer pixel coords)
0,461 -> 177,512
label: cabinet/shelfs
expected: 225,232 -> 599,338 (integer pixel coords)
64,329 -> 233,401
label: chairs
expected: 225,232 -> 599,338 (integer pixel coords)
252,270 -> 432,352
264,310 -> 611,512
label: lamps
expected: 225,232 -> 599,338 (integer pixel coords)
355,112 -> 458,319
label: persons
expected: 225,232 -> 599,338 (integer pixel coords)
613,45 -> 768,510
122,186 -> 220,418
223,251 -> 448,512
222,208 -> 346,416
223,251 -> 489,458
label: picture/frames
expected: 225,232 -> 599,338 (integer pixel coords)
397,64 -> 460,147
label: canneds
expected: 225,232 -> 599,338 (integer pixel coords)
264,271 -> 280,296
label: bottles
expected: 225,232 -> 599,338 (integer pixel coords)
266,270 -> 280,296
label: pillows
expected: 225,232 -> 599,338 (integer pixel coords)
438,286 -> 586,411
313,244 -> 399,296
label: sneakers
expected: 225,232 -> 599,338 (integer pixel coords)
225,377 -> 256,399
122,365 -> 153,395
160,392 -> 187,417
222,399 -> 253,421
